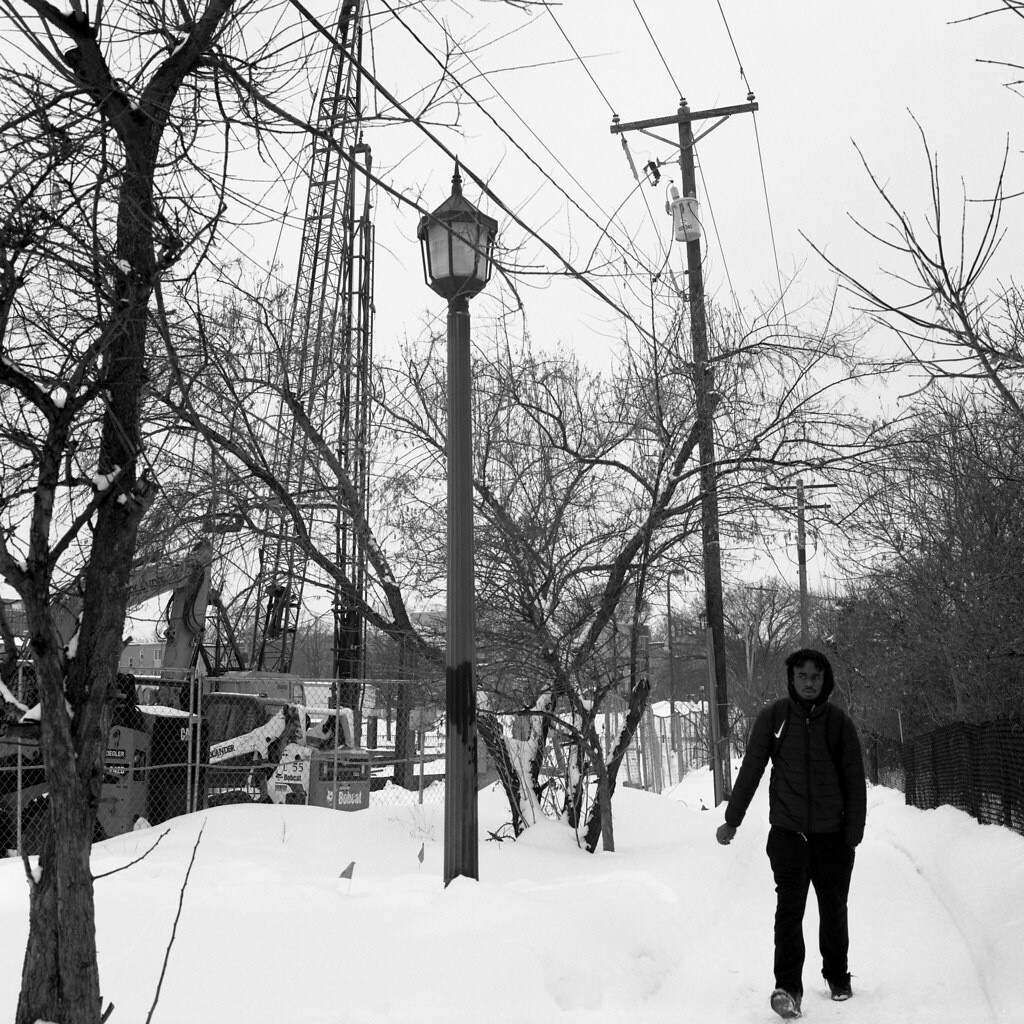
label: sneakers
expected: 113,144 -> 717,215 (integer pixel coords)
771,989 -> 803,1019
827,972 -> 856,1000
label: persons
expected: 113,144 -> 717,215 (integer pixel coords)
318,705 -> 346,750
716,648 -> 866,1020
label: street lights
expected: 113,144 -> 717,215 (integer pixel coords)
413,154 -> 501,888
664,569 -> 686,753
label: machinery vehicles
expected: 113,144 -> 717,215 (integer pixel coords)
0,0 -> 381,857
